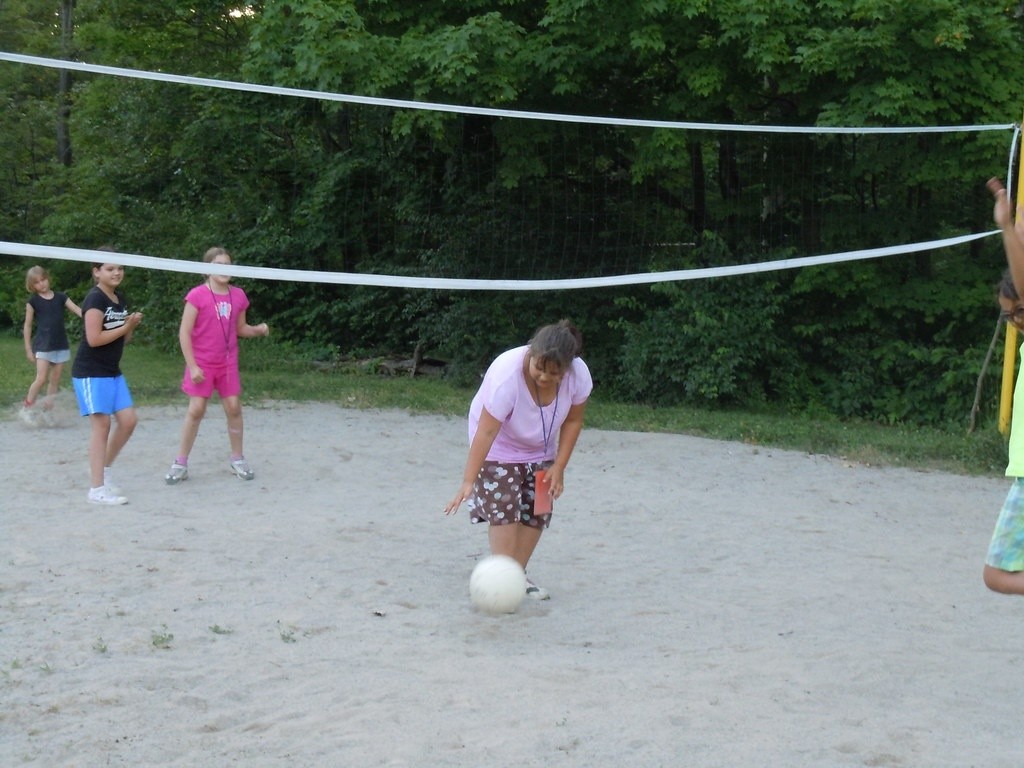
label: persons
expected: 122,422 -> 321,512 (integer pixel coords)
983,177 -> 1024,594
165,246 -> 270,484
443,319 -> 593,600
18,265 -> 82,427
71,246 -> 144,505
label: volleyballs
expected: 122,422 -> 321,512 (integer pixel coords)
469,554 -> 526,613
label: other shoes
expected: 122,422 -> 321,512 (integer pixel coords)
88,489 -> 127,506
19,407 -> 34,425
103,465 -> 120,493
41,407 -> 54,426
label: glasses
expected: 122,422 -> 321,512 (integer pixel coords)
999,304 -> 1024,323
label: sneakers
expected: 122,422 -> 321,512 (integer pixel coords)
525,579 -> 550,600
231,460 -> 254,481
166,463 -> 188,484
